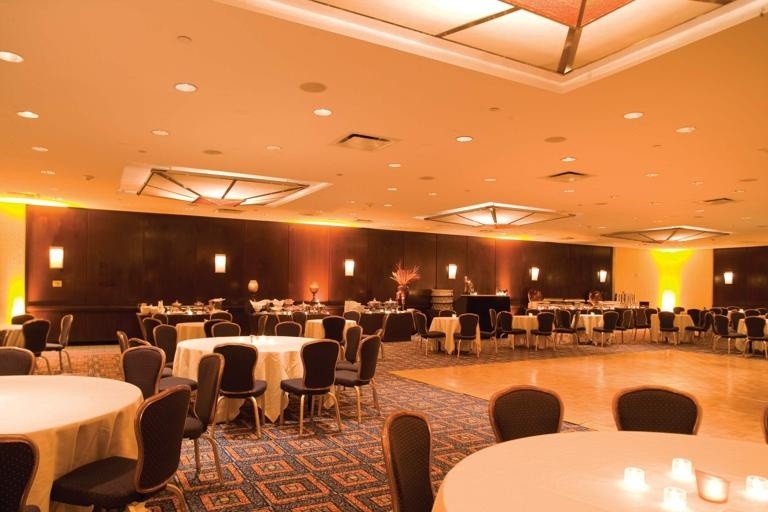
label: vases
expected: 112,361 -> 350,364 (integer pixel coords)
395,285 -> 408,311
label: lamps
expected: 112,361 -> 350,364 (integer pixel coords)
724,271 -> 733,286
48,245 -> 63,270
530,265 -> 540,282
423,202 -> 576,233
137,167 -> 331,211
344,258 -> 355,277
600,226 -> 732,245
447,264 -> 457,280
214,253 -> 227,275
598,265 -> 608,284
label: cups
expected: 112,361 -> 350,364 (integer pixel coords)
624,456 -> 768,512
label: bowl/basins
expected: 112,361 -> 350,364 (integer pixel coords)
247,298 -> 296,314
